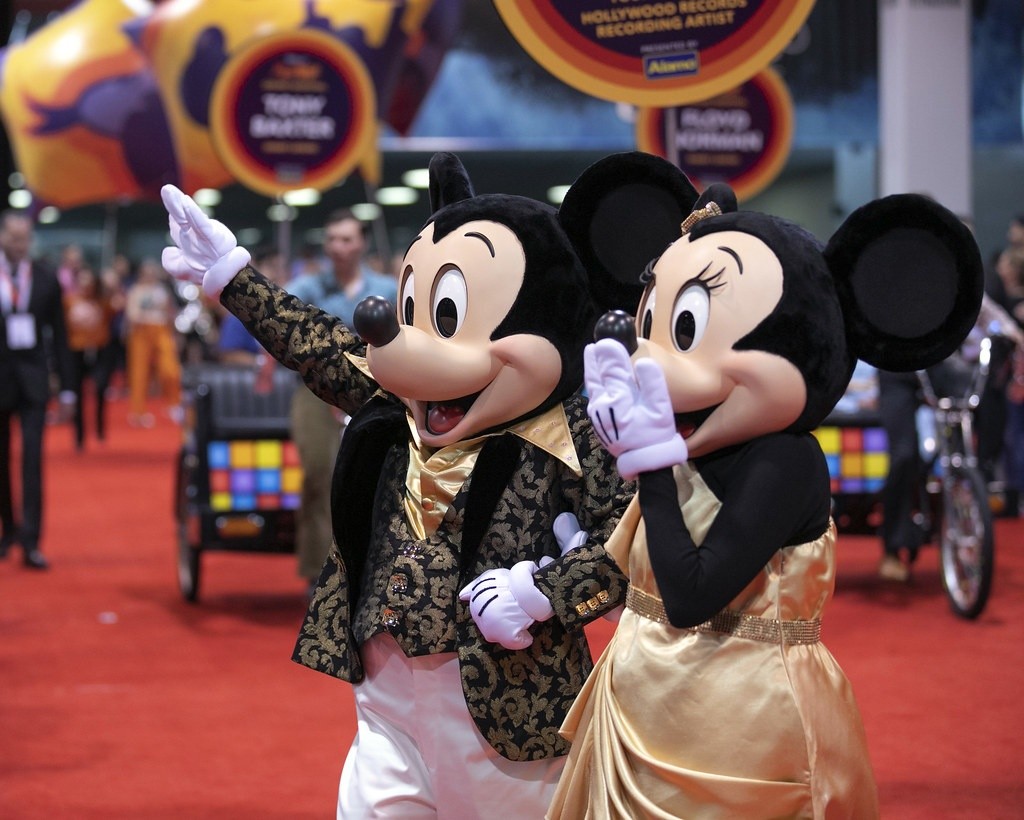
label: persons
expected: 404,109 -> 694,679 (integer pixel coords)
878,213 -> 1024,578
197,210 -> 399,582
0,211 -> 193,566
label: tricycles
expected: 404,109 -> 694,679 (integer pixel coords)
802,331 -> 1017,622
175,363 -> 351,606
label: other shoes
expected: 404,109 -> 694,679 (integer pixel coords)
24,546 -> 47,569
880,550 -> 907,580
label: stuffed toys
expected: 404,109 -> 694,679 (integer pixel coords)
535,151 -> 984,820
159,153 -> 702,819
